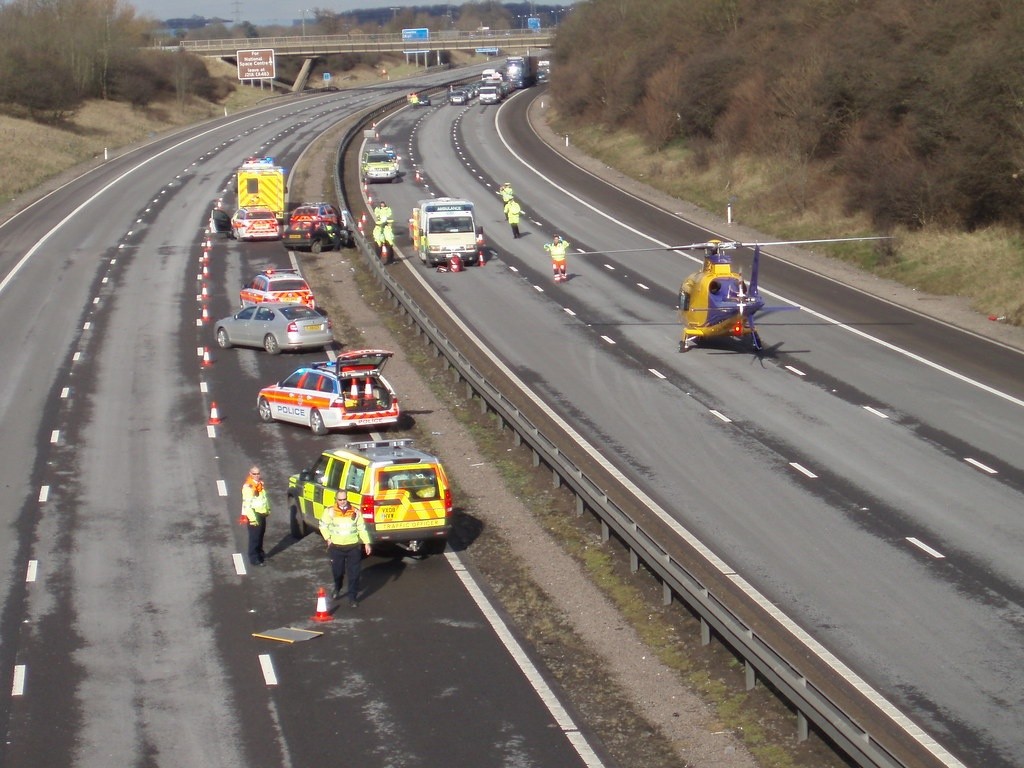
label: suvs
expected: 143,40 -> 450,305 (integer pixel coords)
237,269 -> 316,309
208,206 -> 282,241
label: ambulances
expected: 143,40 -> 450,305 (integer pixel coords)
362,149 -> 399,182
291,445 -> 454,558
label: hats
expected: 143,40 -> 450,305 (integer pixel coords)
505,183 -> 511,188
375,221 -> 382,225
387,220 -> 395,225
509,195 -> 514,200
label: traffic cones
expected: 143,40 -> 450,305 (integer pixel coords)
208,400 -> 223,424
200,238 -> 214,320
310,585 -> 336,623
477,250 -> 486,267
350,378 -> 375,401
475,232 -> 487,248
414,171 -> 423,183
371,122 -> 402,160
353,177 -> 374,234
201,346 -> 216,367
380,244 -> 389,259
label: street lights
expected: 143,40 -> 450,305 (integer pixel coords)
298,9 -> 309,42
519,8 -> 573,35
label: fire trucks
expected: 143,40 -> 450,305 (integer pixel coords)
234,155 -> 289,219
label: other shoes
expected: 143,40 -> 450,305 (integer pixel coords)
256,560 -> 264,567
331,587 -> 339,599
347,596 -> 358,608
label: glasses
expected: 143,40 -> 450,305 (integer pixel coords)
250,471 -> 260,476
336,498 -> 347,501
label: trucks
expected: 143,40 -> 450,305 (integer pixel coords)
505,54 -> 538,89
414,199 -> 480,268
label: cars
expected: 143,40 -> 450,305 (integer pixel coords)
292,201 -> 343,222
282,218 -> 349,254
418,68 -> 514,104
255,349 -> 400,437
214,302 -> 333,355
538,59 -> 551,76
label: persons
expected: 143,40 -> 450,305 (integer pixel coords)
499,182 -> 513,221
544,234 -> 569,283
241,466 -> 270,566
373,201 -> 398,264
319,490 -> 372,607
407,93 -> 418,108
324,224 -> 341,251
504,195 -> 520,238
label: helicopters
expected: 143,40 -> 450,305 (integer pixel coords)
549,231 -> 902,359
